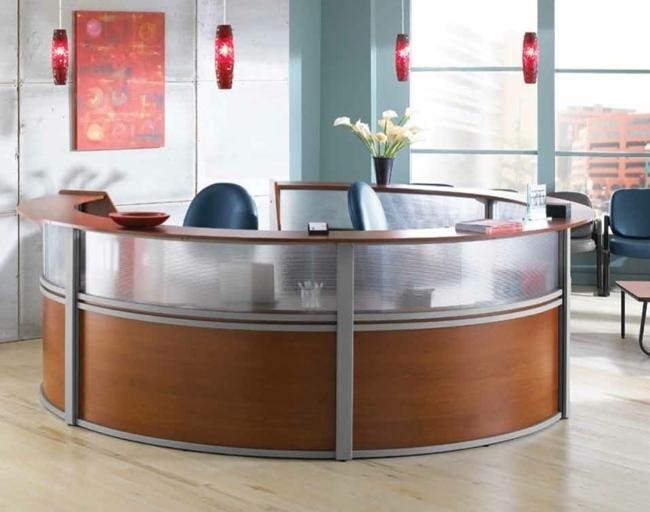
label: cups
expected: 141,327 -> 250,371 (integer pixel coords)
301,288 -> 319,309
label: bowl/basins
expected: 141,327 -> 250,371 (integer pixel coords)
108,210 -> 170,228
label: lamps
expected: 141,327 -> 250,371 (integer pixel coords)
52,1 -> 70,87
395,0 -> 409,82
522,1 -> 539,84
214,0 -> 234,89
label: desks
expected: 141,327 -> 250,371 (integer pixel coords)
614,279 -> 650,356
14,181 -> 604,466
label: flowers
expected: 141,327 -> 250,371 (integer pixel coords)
328,106 -> 425,156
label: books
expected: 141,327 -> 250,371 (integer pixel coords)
455,218 -> 523,236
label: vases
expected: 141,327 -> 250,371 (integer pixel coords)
373,156 -> 395,185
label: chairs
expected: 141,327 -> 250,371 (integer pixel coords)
180,182 -> 258,231
343,178 -> 390,232
546,191 -> 603,297
603,187 -> 650,296
